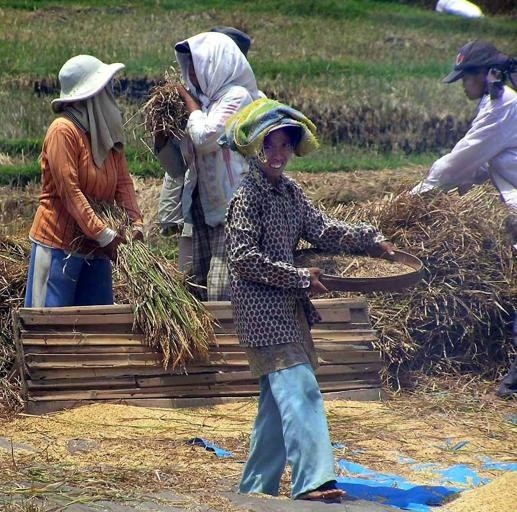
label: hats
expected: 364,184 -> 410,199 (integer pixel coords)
211,25 -> 251,58
52,54 -> 126,116
174,42 -> 191,53
443,41 -> 509,83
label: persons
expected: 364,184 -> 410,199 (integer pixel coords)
209,26 -> 268,101
216,96 -> 395,500
154,31 -> 258,298
24,55 -> 146,310
408,39 -> 517,396
